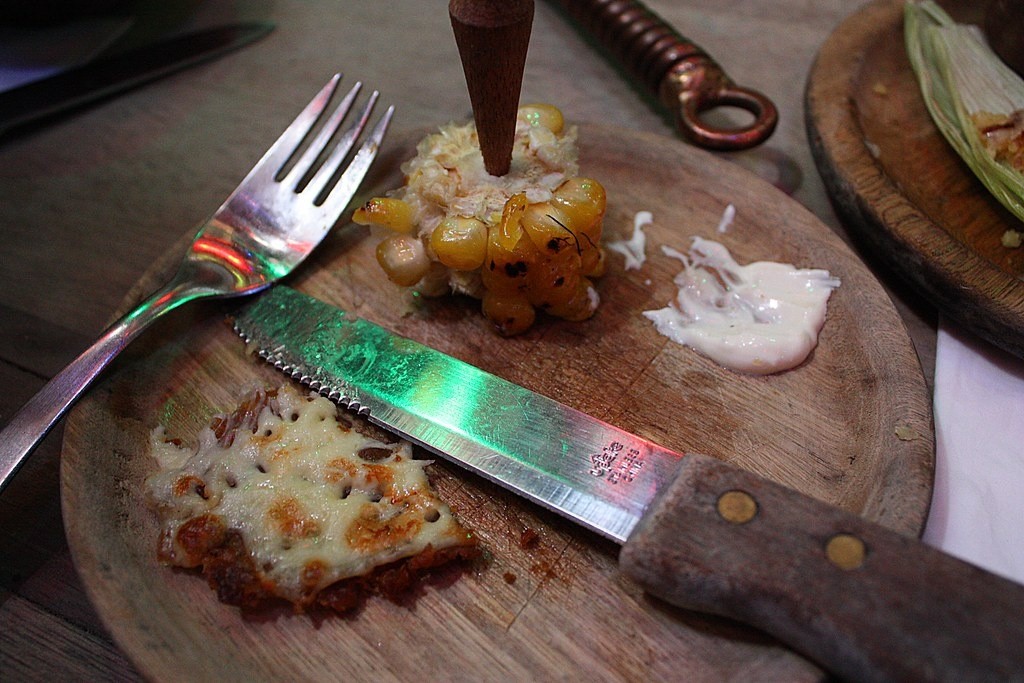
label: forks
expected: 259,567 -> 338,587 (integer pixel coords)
1,68 -> 398,498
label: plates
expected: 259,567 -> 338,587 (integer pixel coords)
806,2 -> 1024,368
56,112 -> 940,683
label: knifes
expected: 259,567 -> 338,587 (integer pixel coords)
225,284 -> 1024,683
0,18 -> 278,141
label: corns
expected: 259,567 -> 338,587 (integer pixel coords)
353,102 -> 607,335
